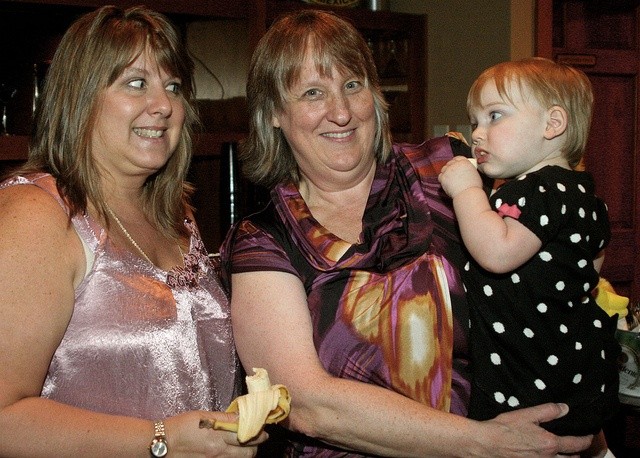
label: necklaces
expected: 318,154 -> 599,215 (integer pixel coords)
102,196 -> 184,270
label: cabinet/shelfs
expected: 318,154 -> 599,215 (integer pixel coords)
1,0 -> 267,256
534,0 -> 640,314
265,2 -> 428,142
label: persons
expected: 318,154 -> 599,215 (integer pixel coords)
438,56 -> 611,452
216,13 -> 604,458
0,5 -> 269,458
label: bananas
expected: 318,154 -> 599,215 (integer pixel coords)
196,368 -> 293,443
467,158 -> 480,168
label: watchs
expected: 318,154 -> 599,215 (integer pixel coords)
150,421 -> 168,457
